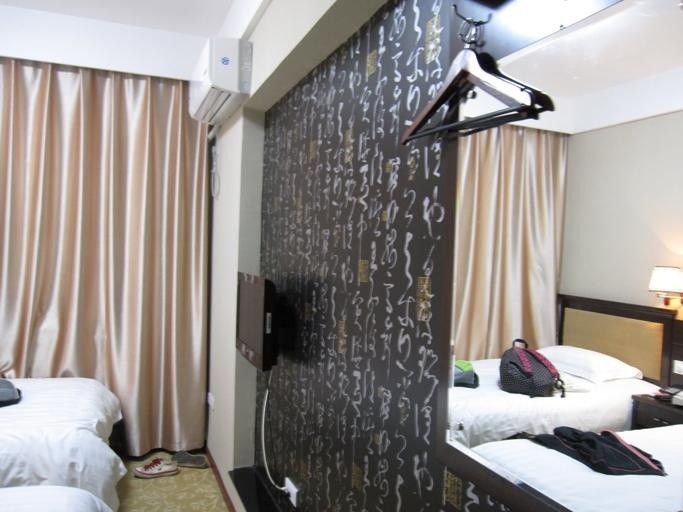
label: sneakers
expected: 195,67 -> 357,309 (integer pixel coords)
172,450 -> 208,468
133,457 -> 179,478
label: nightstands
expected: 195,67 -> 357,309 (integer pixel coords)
628,388 -> 683,431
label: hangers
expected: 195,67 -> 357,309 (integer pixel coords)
397,19 -> 536,146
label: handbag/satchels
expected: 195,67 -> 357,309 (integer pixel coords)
453,360 -> 479,387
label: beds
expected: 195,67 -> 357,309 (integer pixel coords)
448,422 -> 683,511
450,350 -> 664,446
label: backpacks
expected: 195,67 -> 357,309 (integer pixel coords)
500,338 -> 565,397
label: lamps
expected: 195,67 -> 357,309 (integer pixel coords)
648,266 -> 683,308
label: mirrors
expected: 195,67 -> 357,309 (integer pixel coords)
435,1 -> 683,512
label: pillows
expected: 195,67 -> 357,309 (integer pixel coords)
532,344 -> 645,385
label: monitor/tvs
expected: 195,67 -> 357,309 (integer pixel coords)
234,271 -> 282,373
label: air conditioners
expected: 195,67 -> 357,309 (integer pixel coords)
188,36 -> 252,127
